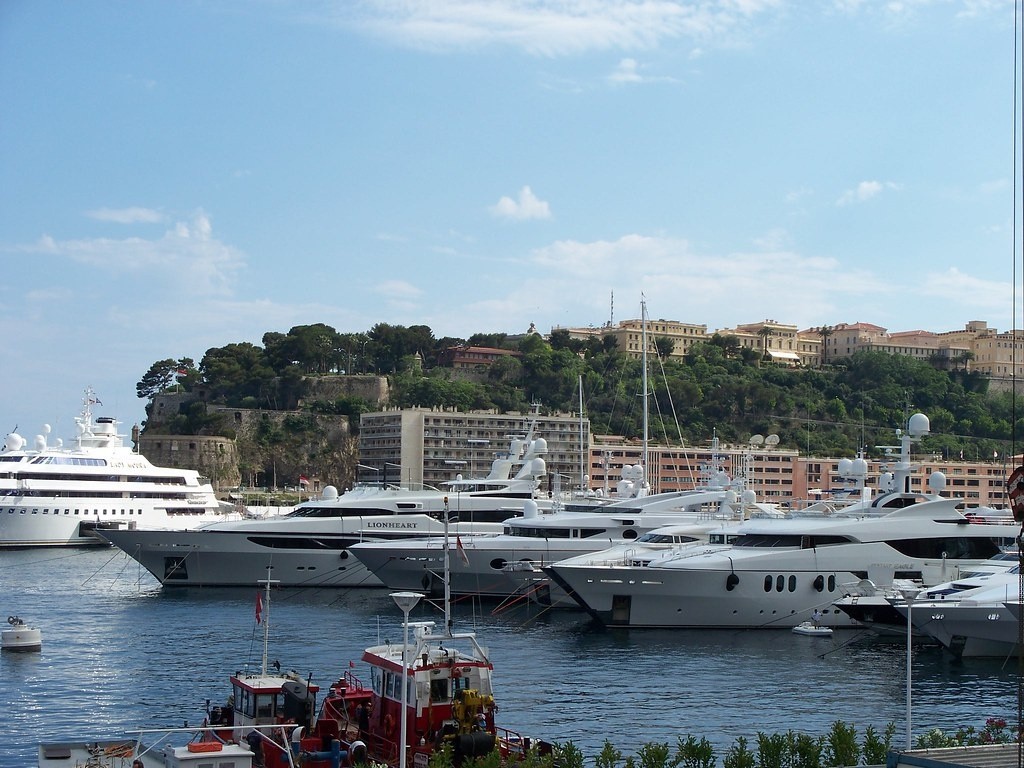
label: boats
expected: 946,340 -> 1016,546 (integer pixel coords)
0,386 -> 243,548
90,291 -> 1024,665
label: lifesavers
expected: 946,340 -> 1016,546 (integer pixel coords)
382,713 -> 395,736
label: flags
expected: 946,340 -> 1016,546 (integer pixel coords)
350,660 -> 355,668
457,536 -> 470,568
177,369 -> 187,377
255,592 -> 262,624
300,475 -> 310,485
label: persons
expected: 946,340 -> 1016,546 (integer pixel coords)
811,608 -> 823,630
355,703 -> 373,740
344,487 -> 350,494
246,724 -> 266,766
348,740 -> 371,768
291,726 -> 307,768
133,760 -> 144,768
